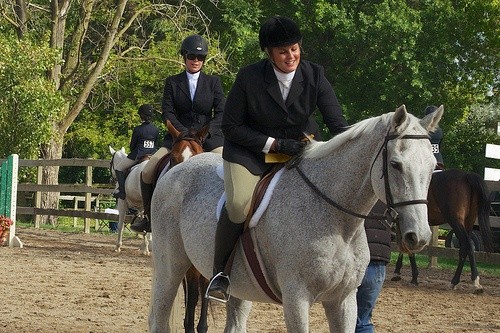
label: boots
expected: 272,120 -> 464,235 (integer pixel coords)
209,200 -> 246,300
132,171 -> 153,233
114,169 -> 126,200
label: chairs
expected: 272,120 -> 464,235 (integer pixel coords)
96,202 -> 131,232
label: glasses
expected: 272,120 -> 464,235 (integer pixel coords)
184,53 -> 205,61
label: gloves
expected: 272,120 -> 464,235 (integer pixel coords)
271,139 -> 301,156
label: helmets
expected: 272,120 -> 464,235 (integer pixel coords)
137,104 -> 152,115
180,36 -> 209,55
259,17 -> 301,52
426,105 -> 439,115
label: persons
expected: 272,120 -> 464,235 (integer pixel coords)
355,199 -> 391,333
208,15 -> 348,300
113,104 -> 158,200
130,35 -> 225,231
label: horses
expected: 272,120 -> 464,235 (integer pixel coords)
388,168 -> 496,295
109,105 -> 444,333
